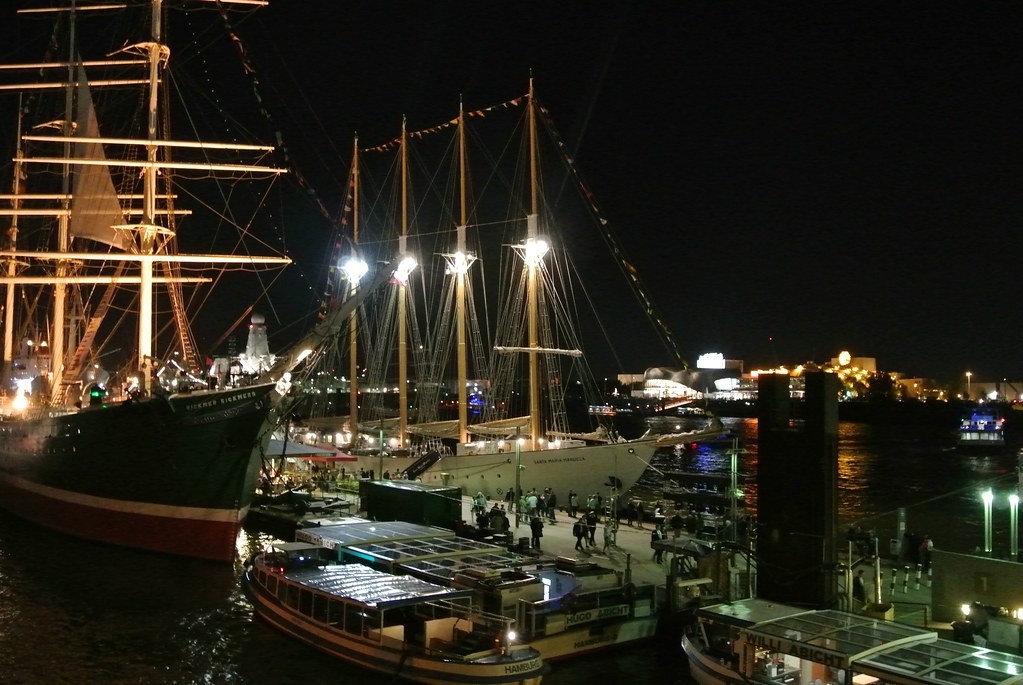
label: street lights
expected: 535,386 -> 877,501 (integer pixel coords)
980,487 -> 997,553
1006,493 -> 1020,556
966,371 -> 973,397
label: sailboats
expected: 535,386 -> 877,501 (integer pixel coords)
0,0 -> 1023,684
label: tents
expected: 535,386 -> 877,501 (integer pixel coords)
297,444 -> 360,477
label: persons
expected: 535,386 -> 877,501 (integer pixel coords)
853,569 -> 872,604
660,525 -> 669,562
568,489 -> 580,517
530,518 -> 544,549
475,503 -> 511,533
383,469 -> 390,479
312,464 -> 319,474
627,498 -> 635,526
636,501 -> 644,527
572,511 -> 620,554
586,492 -> 602,519
474,492 -> 488,507
651,524 -> 663,564
359,467 -> 375,480
670,512 -> 684,538
695,513 -> 704,540
685,515 -> 696,537
504,485 -> 557,525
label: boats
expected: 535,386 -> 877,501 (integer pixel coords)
238,536 -> 552,684
678,594 -> 1023,684
